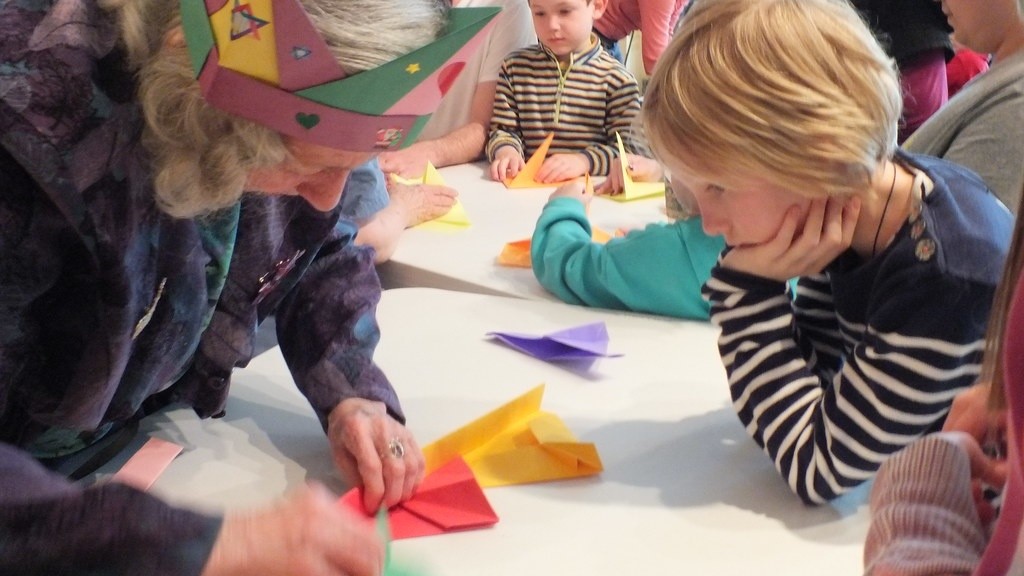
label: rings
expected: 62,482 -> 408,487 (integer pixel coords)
381,439 -> 406,460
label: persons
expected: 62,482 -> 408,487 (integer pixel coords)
485,1 -> 653,186
377,0 -> 538,180
589,1 -> 691,86
944,26 -> 994,102
633,1 -> 1016,504
849,0 -> 956,147
580,1 -> 731,196
890,0 -> 1023,223
861,184 -> 1023,576
531,162 -> 802,321
1,0 -> 427,575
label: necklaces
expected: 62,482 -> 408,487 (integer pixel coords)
871,164 -> 897,261
